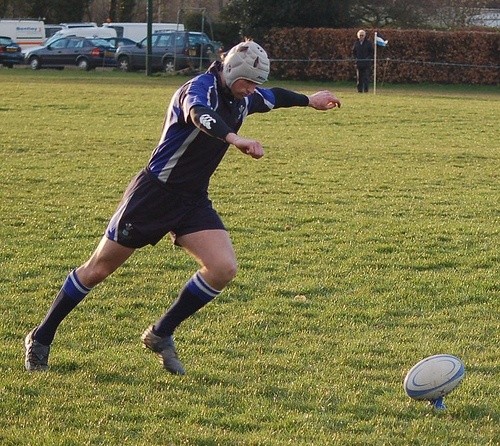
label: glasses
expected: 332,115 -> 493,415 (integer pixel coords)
360,35 -> 364,36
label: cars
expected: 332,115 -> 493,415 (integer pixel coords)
0,19 -> 184,71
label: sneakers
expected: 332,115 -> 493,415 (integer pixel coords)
141,324 -> 185,376
24,326 -> 51,372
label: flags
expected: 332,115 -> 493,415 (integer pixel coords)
375,36 -> 388,47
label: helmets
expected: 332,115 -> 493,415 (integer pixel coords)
223,41 -> 270,89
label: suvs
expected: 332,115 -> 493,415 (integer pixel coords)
114,29 -> 223,73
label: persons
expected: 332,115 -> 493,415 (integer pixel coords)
352,30 -> 374,92
23,36 -> 342,373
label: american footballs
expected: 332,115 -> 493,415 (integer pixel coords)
405,353 -> 465,401
